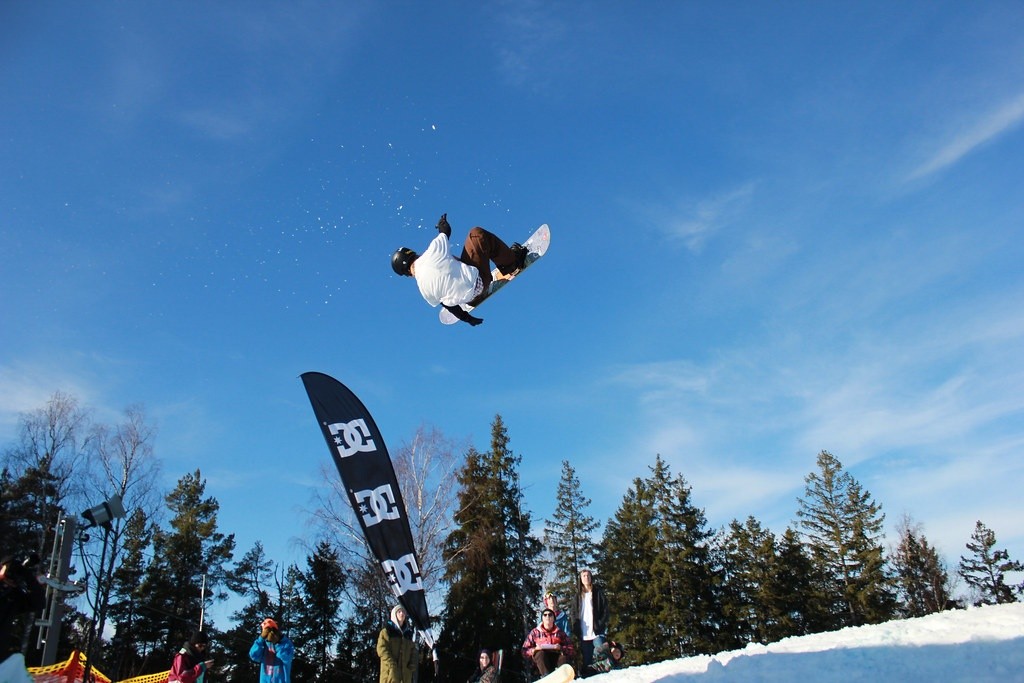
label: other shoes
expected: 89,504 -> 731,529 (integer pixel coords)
505,244 -> 519,273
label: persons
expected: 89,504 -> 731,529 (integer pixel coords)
377,605 -> 417,683
250,619 -> 294,683
391,213 -> 528,327
168,630 -> 214,683
468,568 -> 625,683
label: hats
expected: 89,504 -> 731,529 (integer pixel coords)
610,642 -> 625,659
260,619 -> 278,630
191,631 -> 208,644
390,604 -> 407,635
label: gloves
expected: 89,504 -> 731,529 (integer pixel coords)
458,312 -> 483,326
435,214 -> 452,238
261,627 -> 271,639
267,632 -> 277,644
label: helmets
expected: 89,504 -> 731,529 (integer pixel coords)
391,246 -> 415,275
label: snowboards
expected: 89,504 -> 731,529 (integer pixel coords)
439,224 -> 550,325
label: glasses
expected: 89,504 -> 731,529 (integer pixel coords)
198,643 -> 206,648
543,613 -> 553,617
